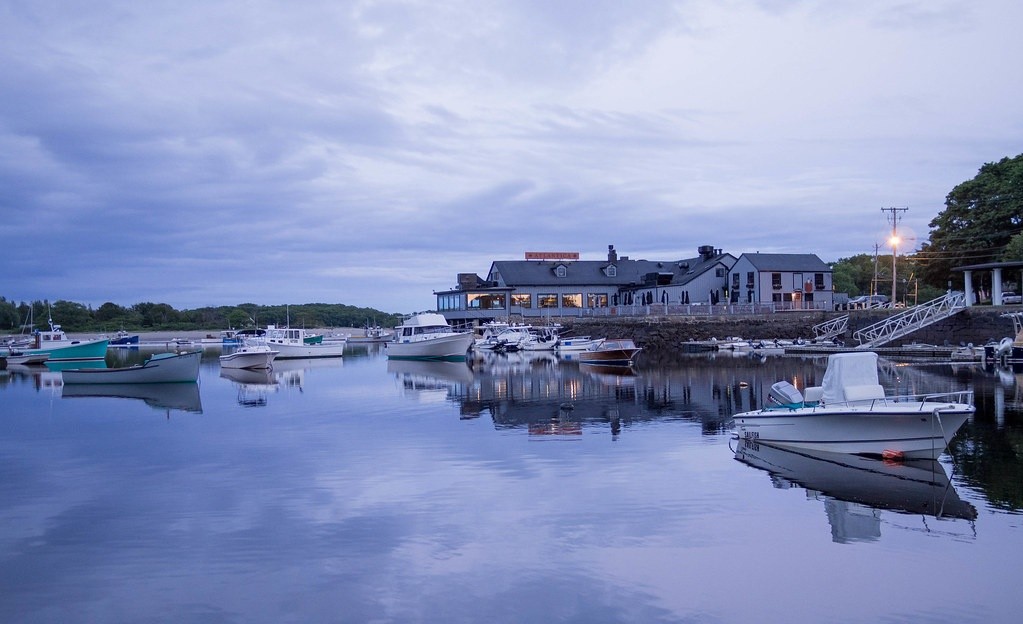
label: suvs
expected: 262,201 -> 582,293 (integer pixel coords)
848,295 -> 889,309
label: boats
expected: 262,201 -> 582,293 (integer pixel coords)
62,350 -> 200,384
219,329 -> 279,369
685,336 -> 833,350
61,382 -> 204,417
476,321 -> 565,351
219,365 -> 278,406
5,353 -> 50,364
950,329 -> 1023,365
730,351 -> 978,459
557,335 -> 642,363
348,316 -> 394,340
384,313 -> 475,359
248,265 -> 347,358
731,441 -> 977,544
386,356 -> 475,389
0,300 -> 139,360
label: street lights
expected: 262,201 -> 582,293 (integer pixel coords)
875,237 -> 897,295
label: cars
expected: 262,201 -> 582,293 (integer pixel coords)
835,296 -> 862,310
1001,291 -> 1022,305
